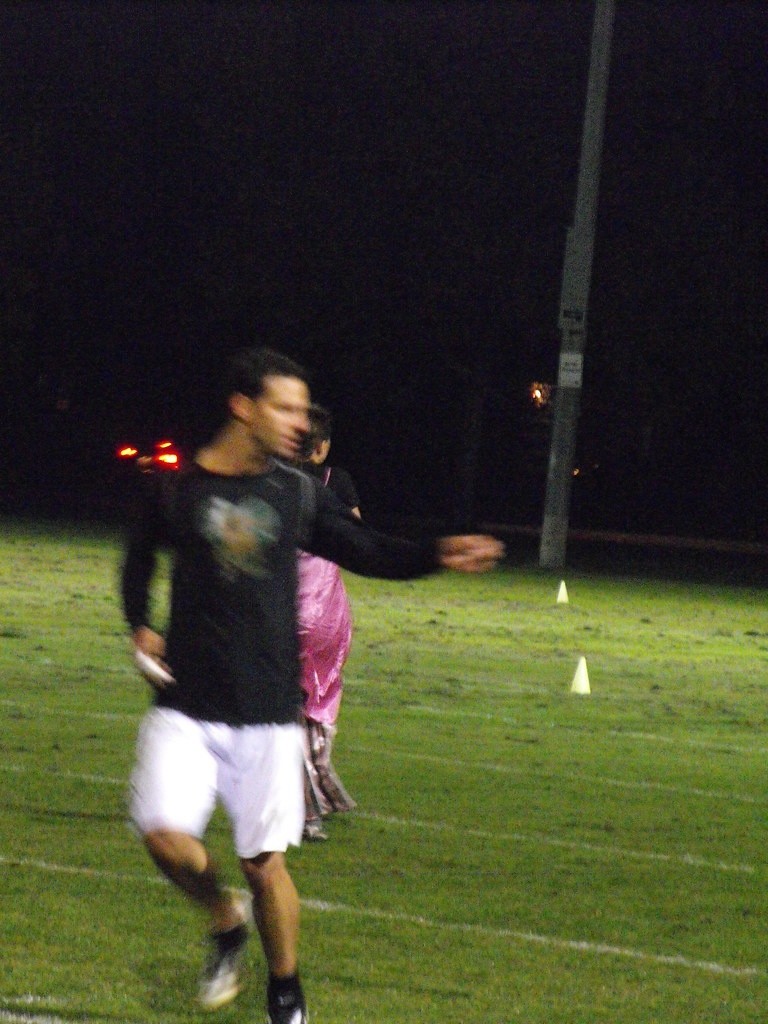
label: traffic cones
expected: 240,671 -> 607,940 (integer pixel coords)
556,579 -> 569,603
569,654 -> 591,695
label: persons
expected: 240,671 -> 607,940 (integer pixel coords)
118,354 -> 509,1024
282,400 -> 363,842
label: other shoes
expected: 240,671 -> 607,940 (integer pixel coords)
199,923 -> 250,1012
266,1000 -> 308,1024
